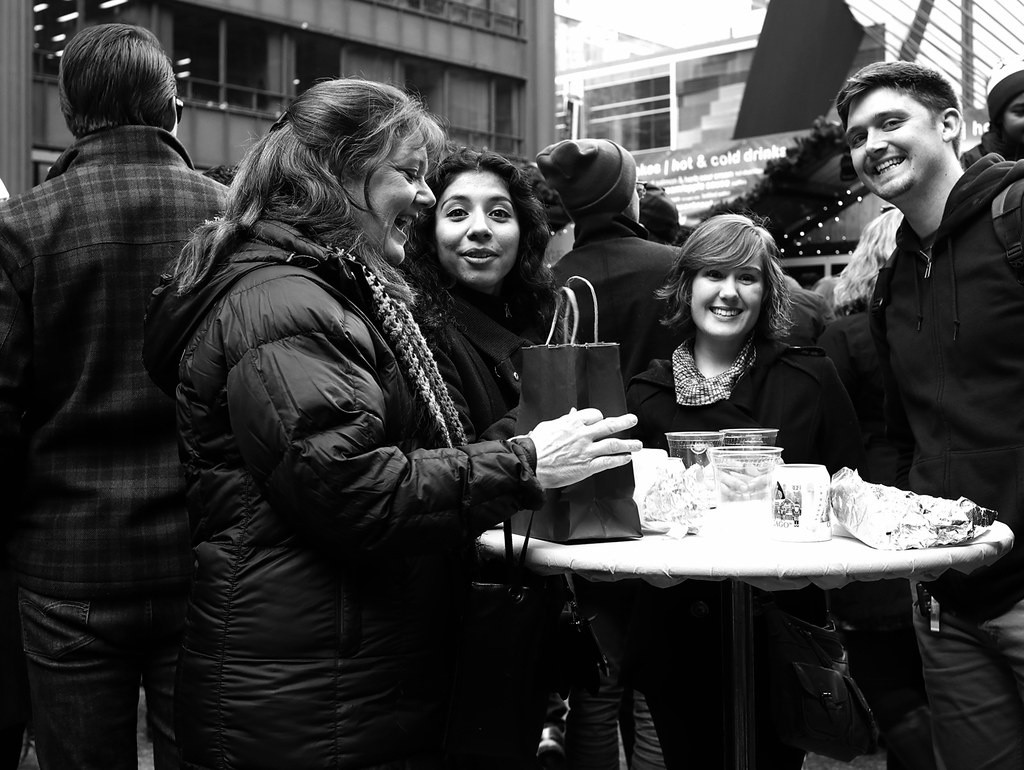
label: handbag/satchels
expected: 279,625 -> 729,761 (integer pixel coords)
511,276 -> 643,543
578,598 -> 736,703
764,606 -> 880,764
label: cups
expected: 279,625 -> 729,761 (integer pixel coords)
663,428 -> 784,529
767,462 -> 833,543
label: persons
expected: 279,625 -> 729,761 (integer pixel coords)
610,216 -> 869,770
538,138 -> 693,386
141,76 -> 642,770
962,56 -> 1023,169
0,23 -> 234,770
520,165 -> 575,267
405,151 -> 577,445
821,208 -> 935,770
638,181 -> 681,245
837,62 -> 1024,769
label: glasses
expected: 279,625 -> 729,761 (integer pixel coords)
174,99 -> 184,123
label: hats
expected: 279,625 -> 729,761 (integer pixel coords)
534,138 -> 637,225
986,54 -> 1024,117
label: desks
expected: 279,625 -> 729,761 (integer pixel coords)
474,494 -> 1014,770
